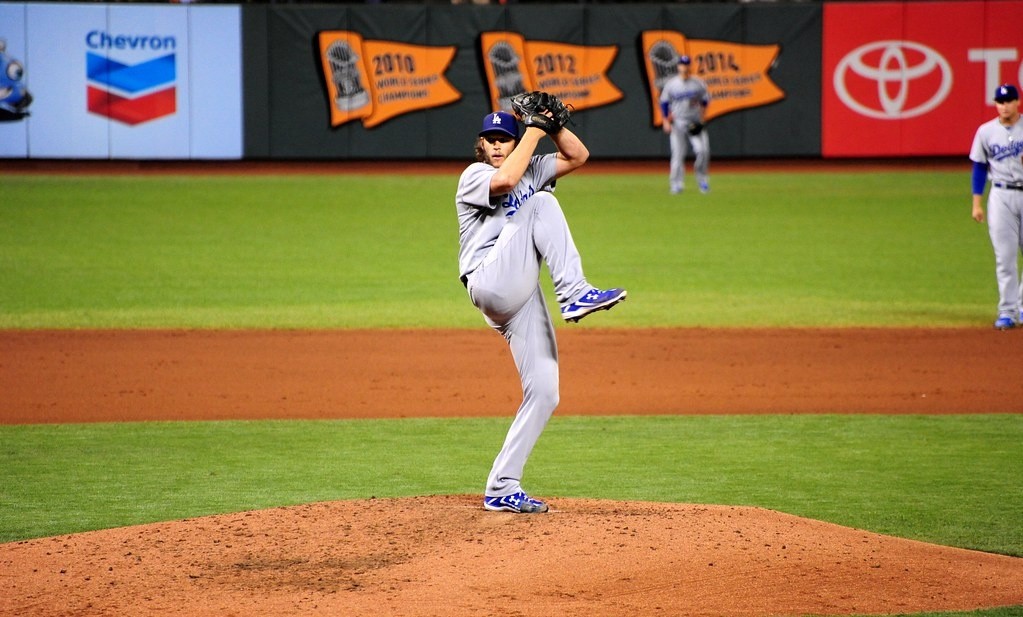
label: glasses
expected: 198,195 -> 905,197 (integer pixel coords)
995,97 -> 1018,104
480,137 -> 514,144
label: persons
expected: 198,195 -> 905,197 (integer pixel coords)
969,84 -> 1023,330
455,91 -> 628,514
659,55 -> 710,194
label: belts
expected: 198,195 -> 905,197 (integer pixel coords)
995,184 -> 1023,191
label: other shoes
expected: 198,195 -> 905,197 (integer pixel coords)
672,185 -> 682,193
699,182 -> 709,192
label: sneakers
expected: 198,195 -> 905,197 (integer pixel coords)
484,491 -> 548,513
1018,308 -> 1023,324
561,289 -> 627,323
995,311 -> 1015,329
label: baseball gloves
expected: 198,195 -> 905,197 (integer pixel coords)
689,121 -> 703,135
510,92 -> 571,136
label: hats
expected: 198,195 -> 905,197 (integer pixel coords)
479,111 -> 519,137
995,85 -> 1018,98
679,56 -> 690,64
